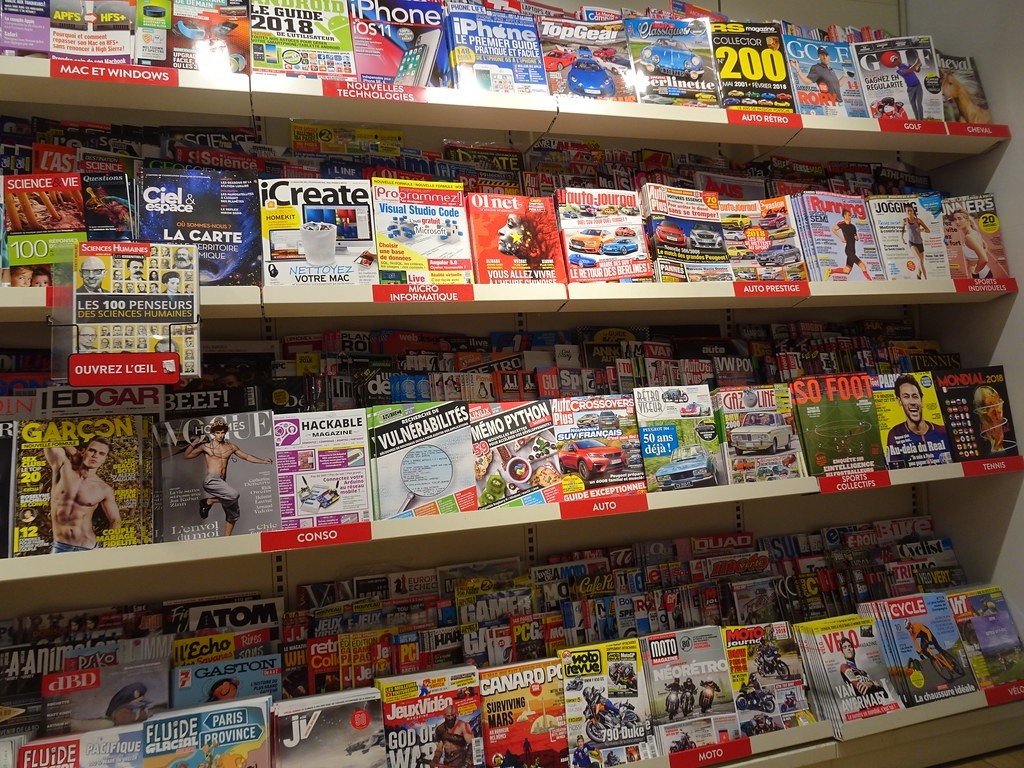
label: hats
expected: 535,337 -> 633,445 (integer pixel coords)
105,682 -> 152,717
890,55 -> 898,63
817,47 -> 829,55
210,416 -> 228,434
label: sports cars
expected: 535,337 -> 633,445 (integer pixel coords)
680,401 -> 710,417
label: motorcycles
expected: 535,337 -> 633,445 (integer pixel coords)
582,685 -> 641,743
661,677 -> 720,754
735,649 -> 802,738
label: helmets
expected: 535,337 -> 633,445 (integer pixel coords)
904,619 -> 910,630
686,678 -> 692,683
583,687 -> 593,697
707,677 -> 713,686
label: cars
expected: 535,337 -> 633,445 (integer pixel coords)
655,443 -> 719,491
558,438 -> 628,480
561,204 -> 639,268
655,220 -> 688,248
729,411 -> 793,454
641,39 -> 705,81
598,410 -> 621,431
695,92 -> 716,104
721,208 -> 801,266
543,44 -> 618,98
576,413 -> 599,428
688,224 -> 723,250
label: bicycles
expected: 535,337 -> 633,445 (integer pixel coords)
916,640 -> 967,682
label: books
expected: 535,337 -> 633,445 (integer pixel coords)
1,116 -> 1012,286
0,0 -> 994,128
1,298 -> 1024,569
0,513 -> 1024,767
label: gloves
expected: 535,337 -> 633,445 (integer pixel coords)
843,70 -> 849,77
930,641 -> 933,645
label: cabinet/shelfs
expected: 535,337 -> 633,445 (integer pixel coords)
0,55 -> 1024,768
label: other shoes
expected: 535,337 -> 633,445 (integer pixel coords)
199,498 -> 212,520
824,267 -> 831,281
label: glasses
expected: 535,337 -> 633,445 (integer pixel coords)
80,334 -> 97,339
82,268 -> 105,276
